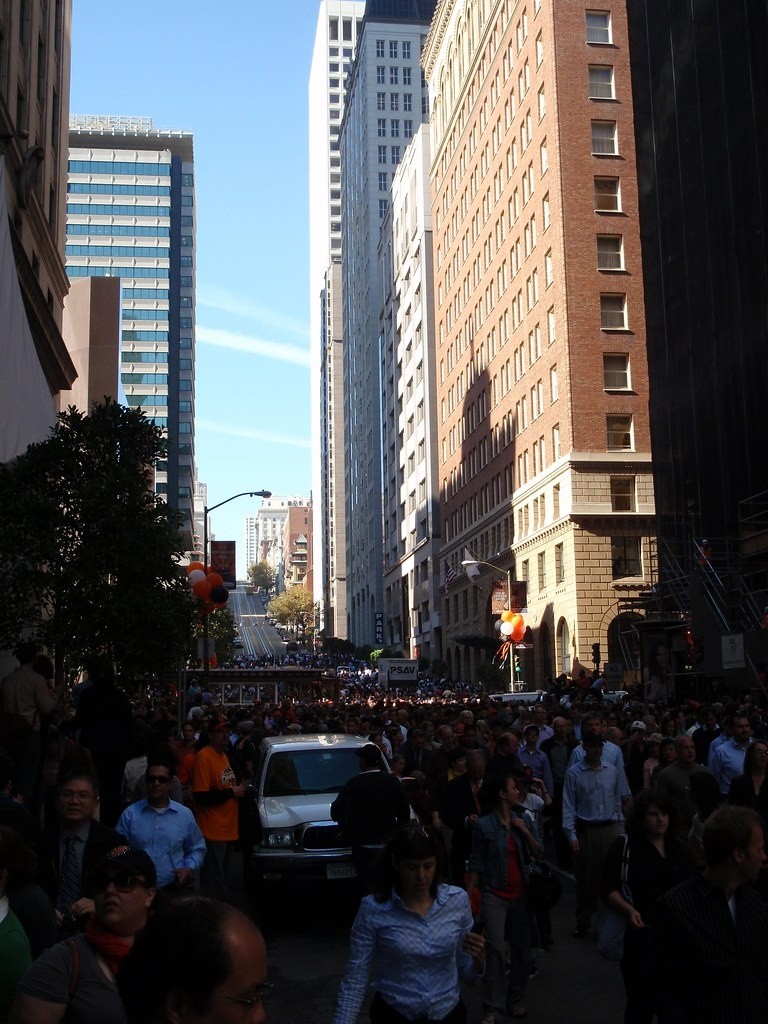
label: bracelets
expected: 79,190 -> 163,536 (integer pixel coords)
542,791 -> 549,795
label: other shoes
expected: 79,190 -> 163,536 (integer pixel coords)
480,1015 -> 497,1024
528,966 -> 539,979
572,925 -> 591,937
511,1006 -> 528,1018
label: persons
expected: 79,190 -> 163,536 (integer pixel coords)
695,539 -> 714,566
0,647 -> 768,1023
758,606 -> 768,639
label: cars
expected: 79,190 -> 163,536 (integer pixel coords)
257,586 -> 299,651
234,639 -> 244,649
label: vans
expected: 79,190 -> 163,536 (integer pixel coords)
242,733 -> 433,919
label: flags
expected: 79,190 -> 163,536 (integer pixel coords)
444,559 -> 457,600
465,548 -> 480,576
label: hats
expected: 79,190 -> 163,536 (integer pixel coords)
512,764 -> 533,781
207,716 -> 229,733
99,845 -> 157,883
356,745 -> 383,764
286,723 -> 302,731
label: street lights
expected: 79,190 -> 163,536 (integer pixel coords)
462,560 -> 515,694
204,489 -> 273,672
301,612 -> 317,652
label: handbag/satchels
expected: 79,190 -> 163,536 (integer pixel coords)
595,894 -> 633,961
0,712 -> 32,753
526,864 -> 562,913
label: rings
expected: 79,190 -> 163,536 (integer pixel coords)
471,946 -> 475,952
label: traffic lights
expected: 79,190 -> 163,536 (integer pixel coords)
514,655 -> 521,673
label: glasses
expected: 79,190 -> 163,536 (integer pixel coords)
528,731 -> 538,736
145,774 -> 170,784
59,789 -> 95,800
94,873 -> 148,894
518,780 -> 533,785
752,750 -> 768,755
210,982 -> 273,1016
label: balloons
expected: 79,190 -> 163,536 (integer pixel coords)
186,562 -> 229,614
495,611 -> 524,642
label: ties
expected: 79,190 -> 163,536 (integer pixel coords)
60,832 -> 80,918
